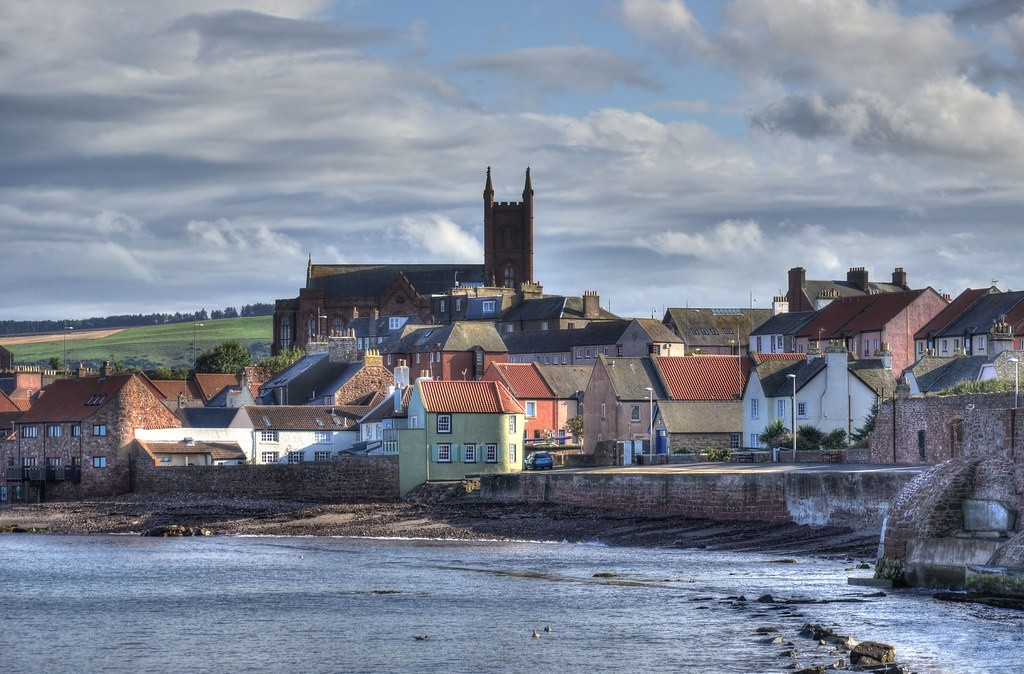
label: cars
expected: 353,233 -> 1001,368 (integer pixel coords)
525,451 -> 554,469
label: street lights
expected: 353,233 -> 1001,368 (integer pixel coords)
11,421 -> 14,436
601,307 -> 610,312
194,323 -> 204,363
686,308 -> 700,355
786,374 -> 796,463
185,353 -> 190,398
728,340 -> 735,354
750,299 -> 758,332
318,316 -> 327,335
645,387 -> 653,462
64,327 -> 73,374
110,354 -> 114,374
308,334 -> 315,346
1007,358 -> 1019,408
696,348 -> 700,353
652,307 -> 656,320
